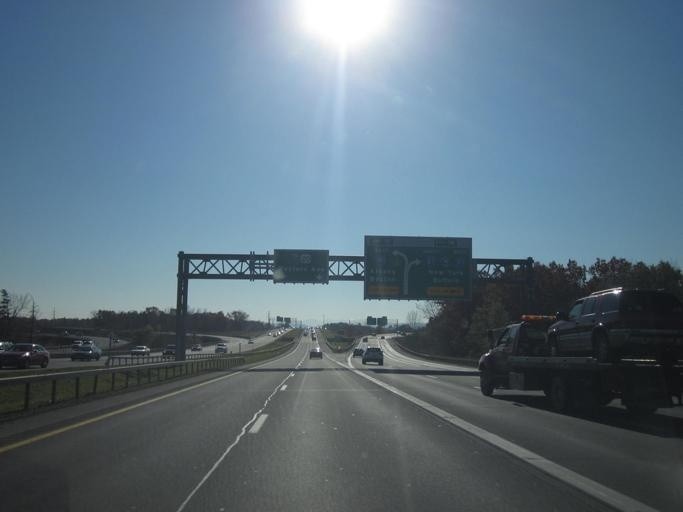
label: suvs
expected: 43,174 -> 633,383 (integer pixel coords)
548,285 -> 682,355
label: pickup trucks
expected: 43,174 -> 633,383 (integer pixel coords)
478,315 -> 671,413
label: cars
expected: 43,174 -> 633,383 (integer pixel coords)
70,337 -> 228,361
0,341 -> 50,369
309,347 -> 384,366
